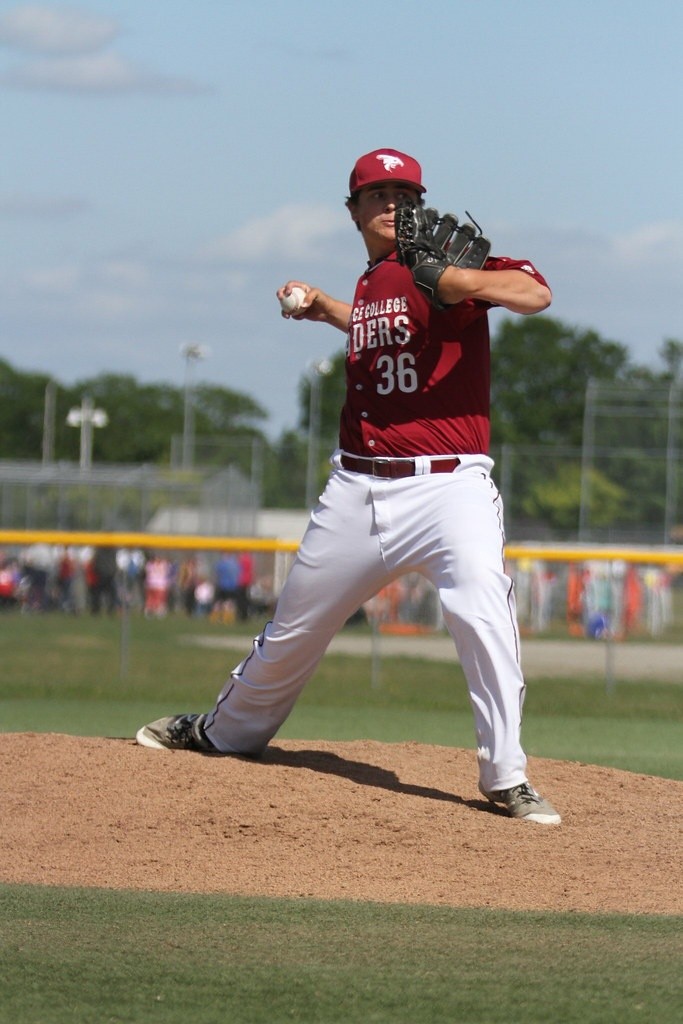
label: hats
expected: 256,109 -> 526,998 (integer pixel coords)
349,149 -> 427,197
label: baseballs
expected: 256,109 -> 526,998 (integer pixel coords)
281,287 -> 308,316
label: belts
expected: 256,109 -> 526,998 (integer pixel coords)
341,454 -> 460,478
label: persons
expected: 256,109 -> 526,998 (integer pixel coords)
180,556 -> 213,614
89,550 -> 121,617
362,573 -> 426,626
142,554 -> 179,617
116,548 -> 147,609
137,149 -> 560,826
0,544 -> 95,614
210,551 -> 253,622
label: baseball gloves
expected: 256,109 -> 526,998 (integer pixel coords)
394,200 -> 492,310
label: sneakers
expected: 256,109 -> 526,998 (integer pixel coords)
136,714 -> 217,751
478,781 -> 560,825
